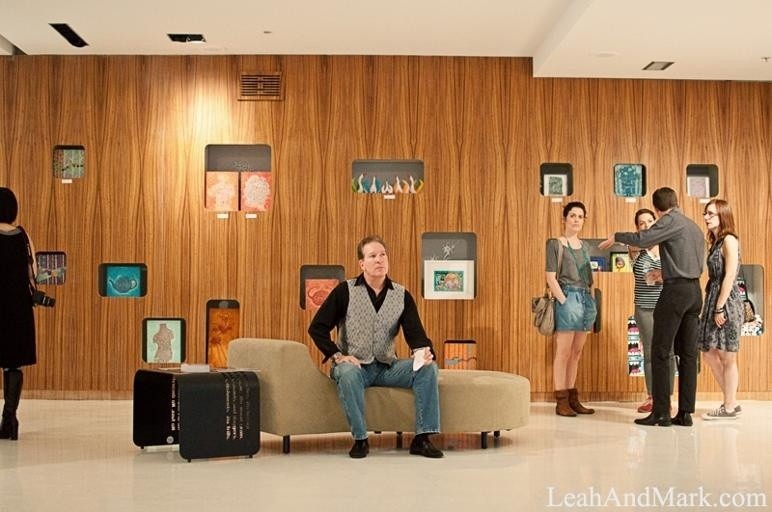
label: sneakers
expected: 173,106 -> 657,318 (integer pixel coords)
721,404 -> 743,415
701,405 -> 737,420
638,396 -> 654,413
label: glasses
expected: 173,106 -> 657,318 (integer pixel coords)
702,210 -> 720,218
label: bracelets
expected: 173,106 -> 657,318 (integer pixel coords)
713,308 -> 724,314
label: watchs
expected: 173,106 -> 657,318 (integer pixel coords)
332,352 -> 342,362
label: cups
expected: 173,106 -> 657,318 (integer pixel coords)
647,275 -> 655,285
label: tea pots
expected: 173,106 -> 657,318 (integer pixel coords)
107,276 -> 137,293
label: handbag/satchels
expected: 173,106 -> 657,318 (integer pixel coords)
533,293 -> 555,336
744,300 -> 756,322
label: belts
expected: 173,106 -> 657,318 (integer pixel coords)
665,278 -> 699,284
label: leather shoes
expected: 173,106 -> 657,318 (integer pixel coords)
635,412 -> 672,426
410,438 -> 443,458
349,439 -> 369,458
671,411 -> 693,426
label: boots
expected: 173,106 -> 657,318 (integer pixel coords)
556,390 -> 577,416
567,389 -> 594,414
0,370 -> 23,440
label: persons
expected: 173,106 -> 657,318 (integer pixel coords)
598,187 -> 705,427
307,235 -> 444,458
550,180 -> 560,194
627,209 -> 672,413
700,199 -> 743,418
0,188 -> 39,440
545,202 -> 595,417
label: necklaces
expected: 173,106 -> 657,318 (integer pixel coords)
649,249 -> 659,257
561,232 -> 594,288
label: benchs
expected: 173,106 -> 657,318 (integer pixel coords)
227,338 -> 531,453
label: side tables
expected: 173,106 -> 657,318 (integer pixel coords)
132,367 -> 260,463
